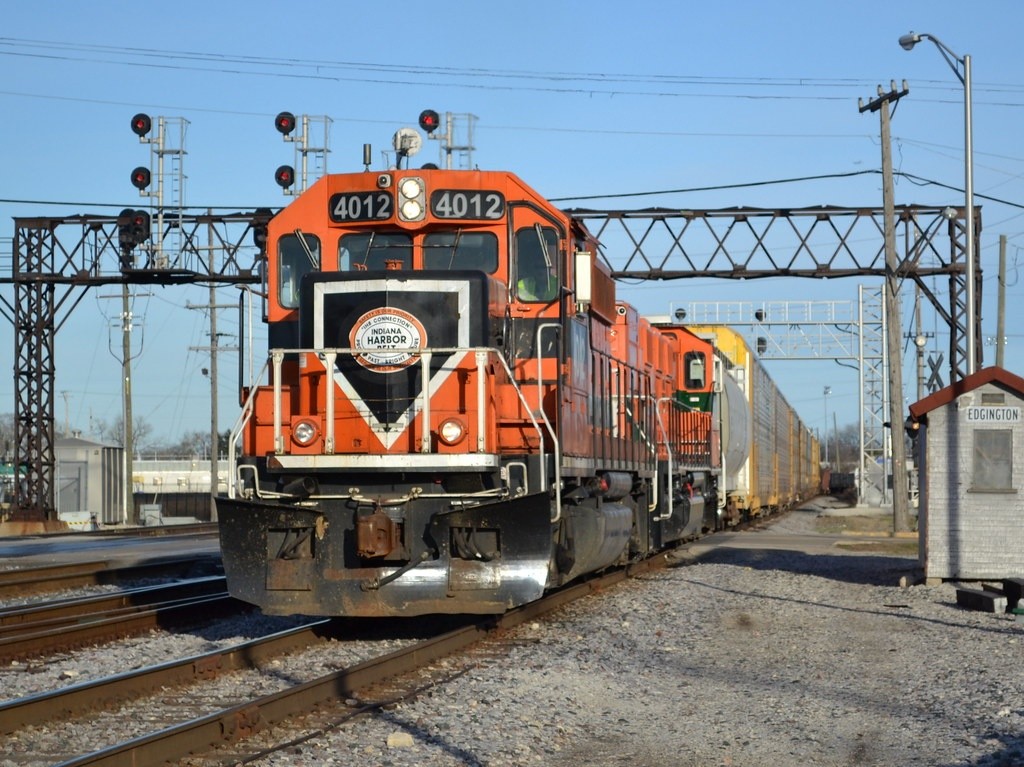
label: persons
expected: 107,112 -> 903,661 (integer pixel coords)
518,254 -> 557,301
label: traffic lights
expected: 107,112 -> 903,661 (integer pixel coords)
275,166 -> 294,185
131,168 -> 150,187
131,114 -> 151,135
418,110 -> 439,130
275,111 -> 294,132
135,210 -> 146,243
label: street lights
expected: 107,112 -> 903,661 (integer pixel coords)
823,386 -> 832,462
898,31 -> 977,378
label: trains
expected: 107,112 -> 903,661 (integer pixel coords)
214,143 -> 821,618
